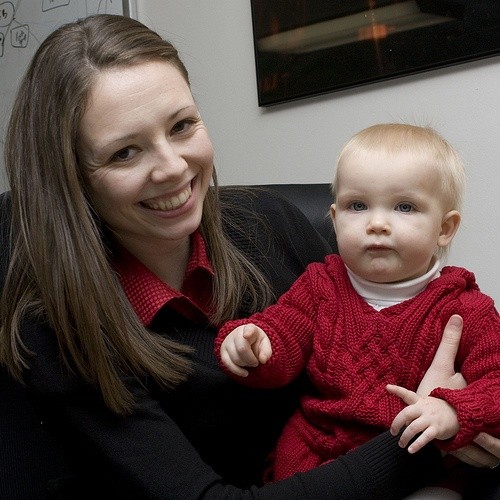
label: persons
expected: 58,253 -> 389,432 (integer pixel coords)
1,13 -> 500,500
215,125 -> 500,479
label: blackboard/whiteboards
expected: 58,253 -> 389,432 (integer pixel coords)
0,0 -> 138,197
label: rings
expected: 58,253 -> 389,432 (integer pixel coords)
490,463 -> 499,469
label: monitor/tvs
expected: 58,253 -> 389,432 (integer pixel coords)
250,0 -> 500,107
